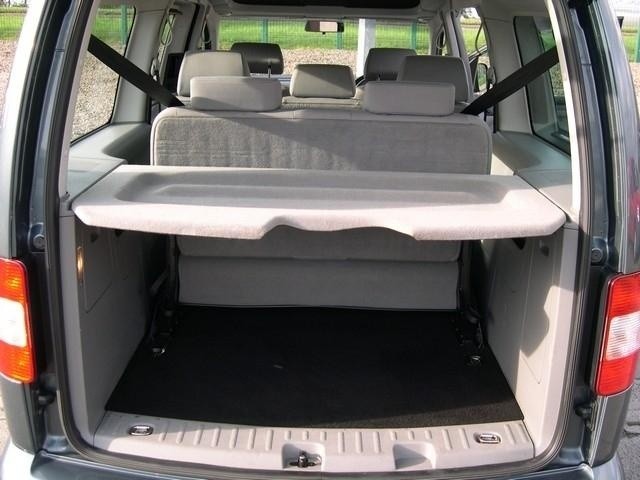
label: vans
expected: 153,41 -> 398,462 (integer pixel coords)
0,0 -> 640,479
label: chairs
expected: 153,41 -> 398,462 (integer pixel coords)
150,40 -> 493,313
